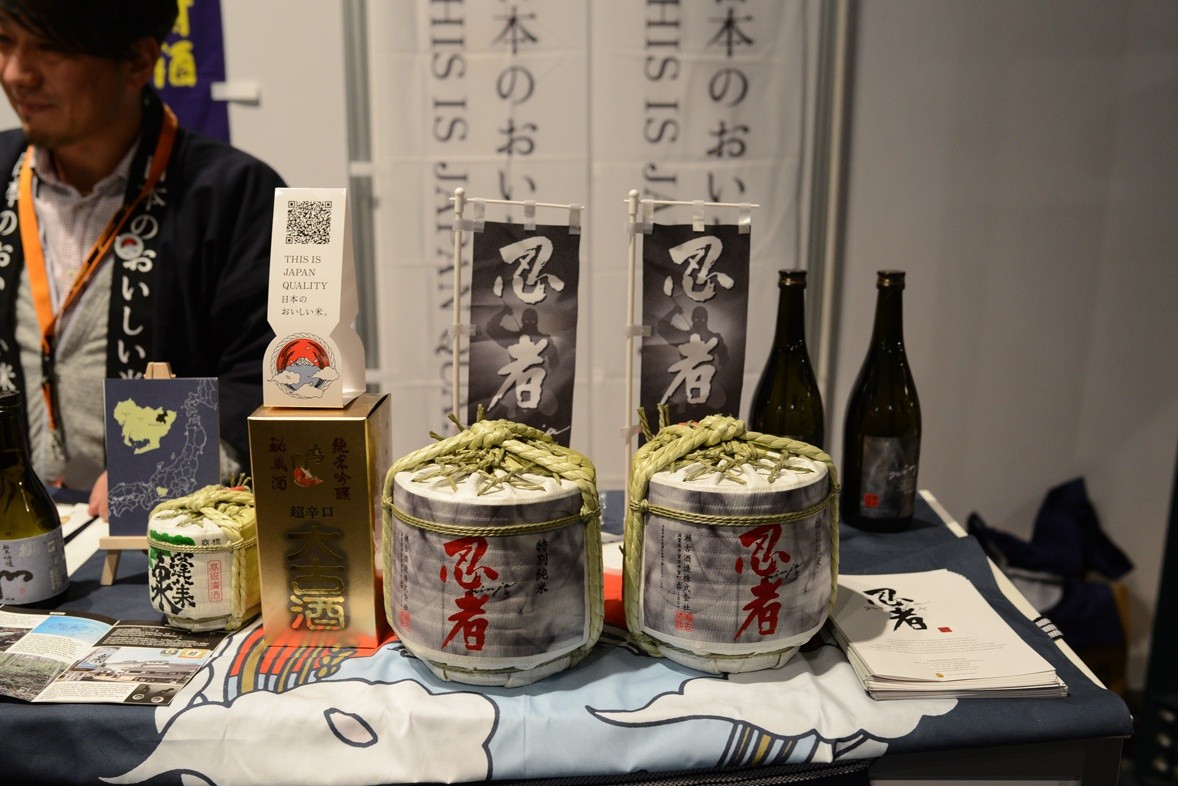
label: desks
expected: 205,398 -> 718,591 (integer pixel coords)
0,482 -> 1134,784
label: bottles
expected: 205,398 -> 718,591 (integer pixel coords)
746,267 -> 824,461
841,267 -> 923,536
2,390 -> 68,612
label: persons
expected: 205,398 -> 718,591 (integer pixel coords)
1,0 -> 290,519
